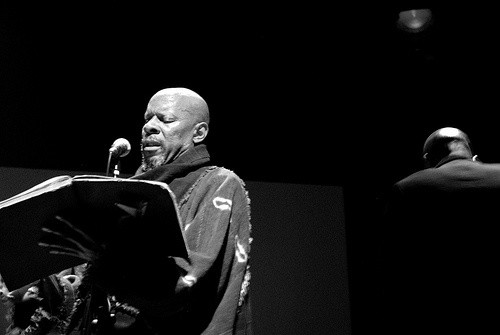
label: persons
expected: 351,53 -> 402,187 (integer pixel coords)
377,126 -> 499,335
126,88 -> 253,334
6,263 -> 86,335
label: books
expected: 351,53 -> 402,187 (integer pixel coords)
0,173 -> 192,293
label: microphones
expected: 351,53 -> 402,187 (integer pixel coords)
109,137 -> 131,159
